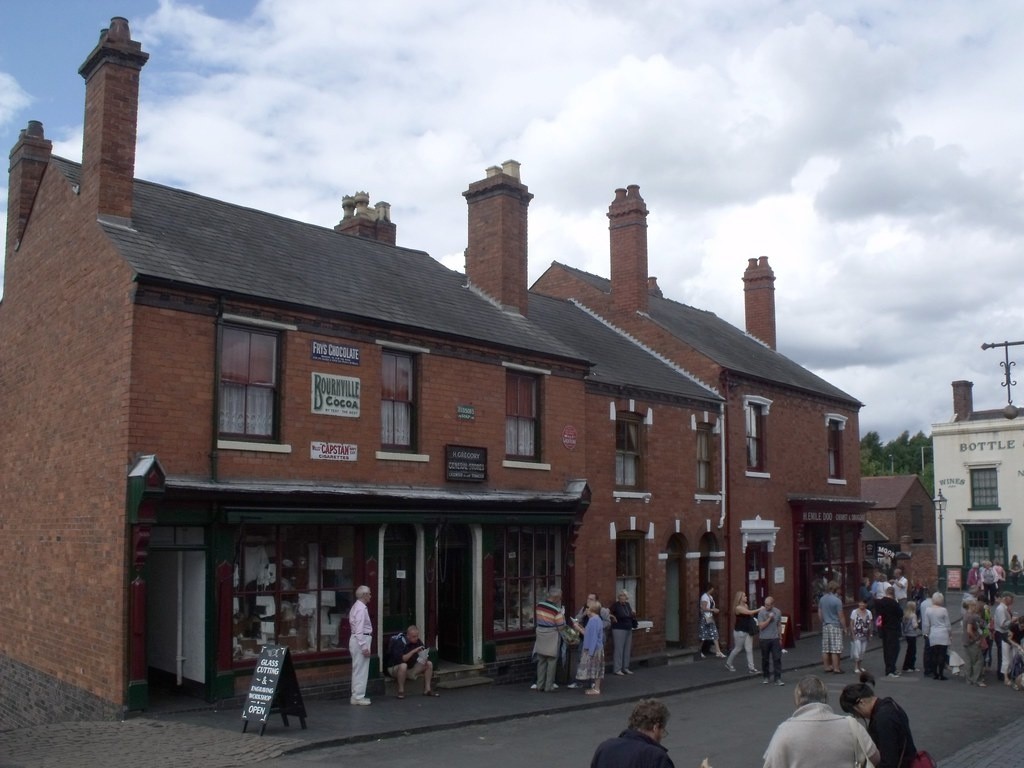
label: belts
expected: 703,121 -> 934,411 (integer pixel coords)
352,633 -> 370,635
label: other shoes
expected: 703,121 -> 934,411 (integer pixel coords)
924,671 -> 947,680
531,681 -> 561,692
397,690 -> 406,699
422,690 -> 440,697
854,667 -> 866,674
615,668 -> 633,675
749,669 -> 758,673
566,682 -> 578,688
966,673 -> 1021,690
886,669 -> 902,678
824,667 -> 846,673
725,664 -> 736,672
762,676 -> 785,686
585,689 -> 601,695
350,697 -> 371,706
902,667 -> 920,672
715,652 -> 727,658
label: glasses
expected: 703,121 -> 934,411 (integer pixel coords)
654,720 -> 669,738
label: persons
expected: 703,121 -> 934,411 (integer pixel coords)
762,674 -> 881,768
962,555 -> 1024,690
529,587 -> 638,695
698,583 -> 727,658
725,590 -> 784,686
384,624 -> 441,699
816,567 -> 952,681
349,585 -> 372,706
590,697 -> 674,768
840,672 -> 918,768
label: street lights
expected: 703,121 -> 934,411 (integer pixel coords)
932,488 -> 948,605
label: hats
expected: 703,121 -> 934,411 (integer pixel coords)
1002,591 -> 1017,597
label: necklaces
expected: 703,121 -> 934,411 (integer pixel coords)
621,603 -> 629,616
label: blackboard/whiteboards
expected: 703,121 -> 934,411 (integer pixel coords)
241,647 -> 308,723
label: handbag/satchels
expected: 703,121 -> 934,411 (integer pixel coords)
705,616 -> 715,624
906,751 -> 937,768
